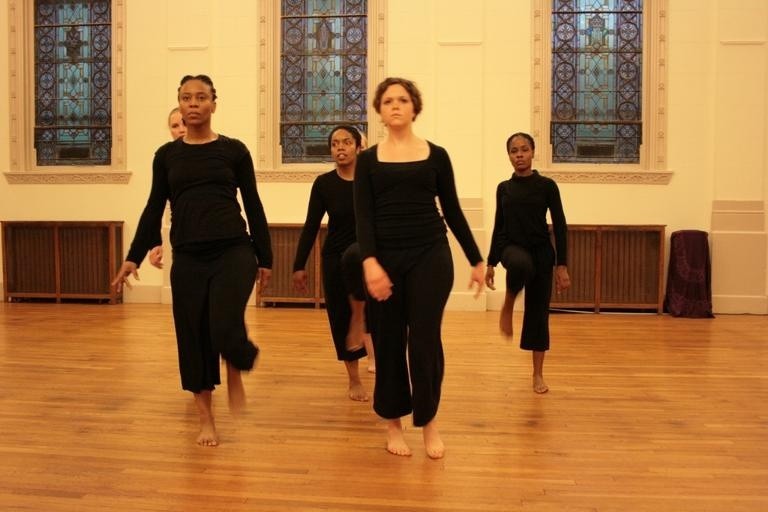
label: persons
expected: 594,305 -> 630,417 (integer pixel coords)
360,131 -> 376,374
292,125 -> 369,403
150,106 -> 186,269
110,74 -> 273,446
485,132 -> 571,394
354,77 -> 484,460
